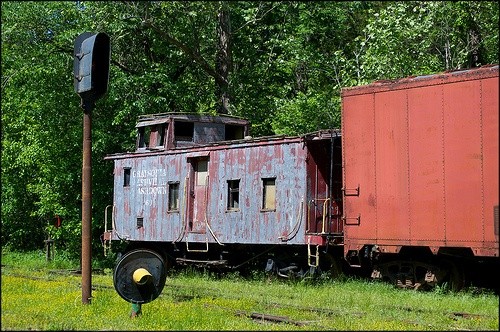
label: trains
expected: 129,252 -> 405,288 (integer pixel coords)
96,63 -> 499,282
